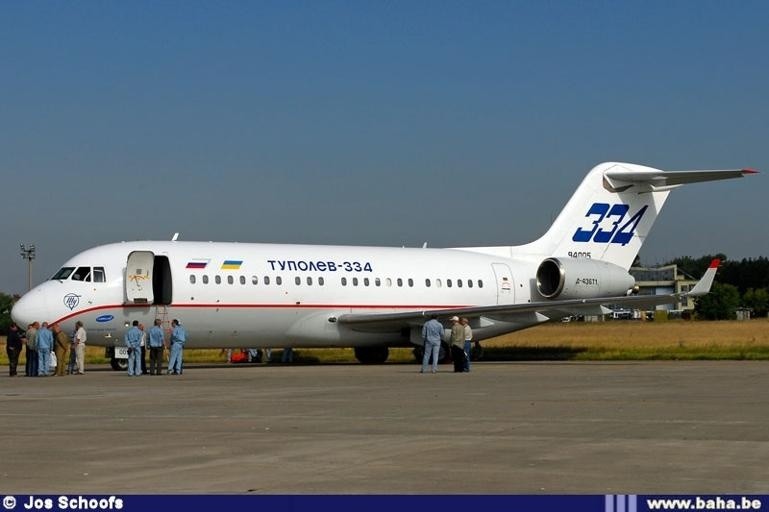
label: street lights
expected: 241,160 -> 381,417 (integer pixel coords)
17,240 -> 37,293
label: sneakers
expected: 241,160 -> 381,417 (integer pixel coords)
128,371 -> 182,376
419,368 -> 427,373
10,370 -> 85,377
453,368 -> 470,373
432,369 -> 440,373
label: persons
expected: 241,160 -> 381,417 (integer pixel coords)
223,348 -> 294,364
462,318 -> 473,372
4,319 -> 88,377
449,317 -> 465,373
419,313 -> 445,373
123,318 -> 188,377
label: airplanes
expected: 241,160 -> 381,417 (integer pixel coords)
9,160 -> 764,370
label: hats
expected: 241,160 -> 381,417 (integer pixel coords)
449,316 -> 459,321
